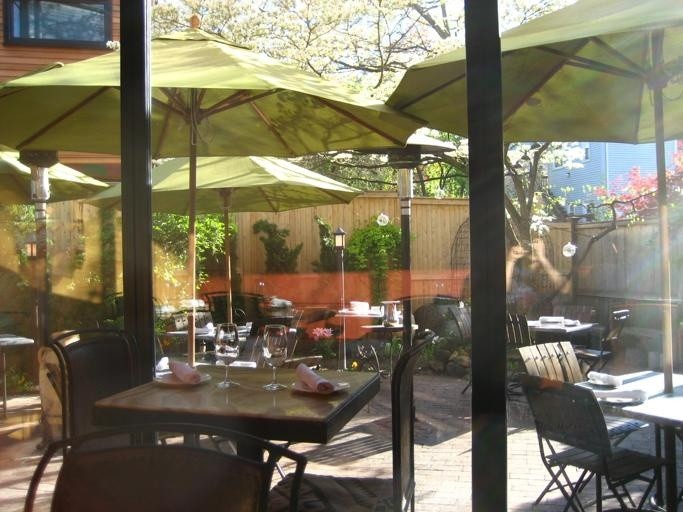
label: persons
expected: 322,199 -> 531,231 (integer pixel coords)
505,227 -> 573,326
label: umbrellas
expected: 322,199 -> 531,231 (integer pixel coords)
78,155 -> 365,332
0,150 -> 111,206
0,14 -> 429,369
386,0 -> 683,512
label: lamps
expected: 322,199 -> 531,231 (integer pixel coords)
26,233 -> 37,259
333,228 -> 346,250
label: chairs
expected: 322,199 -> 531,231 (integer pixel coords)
48,329 -> 143,457
269,349 -> 417,512
165,291 -> 336,369
24,423 -> 307,512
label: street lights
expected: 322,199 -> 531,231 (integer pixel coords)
24,234 -> 38,339
333,228 -> 347,370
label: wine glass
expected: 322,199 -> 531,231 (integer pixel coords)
213,323 -> 241,389
263,324 -> 287,391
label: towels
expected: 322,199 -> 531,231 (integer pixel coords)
169,361 -> 201,385
296,362 -> 333,394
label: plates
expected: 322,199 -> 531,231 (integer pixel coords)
600,393 -> 647,404
153,370 -> 212,387
289,377 -> 351,394
588,377 -> 623,386
